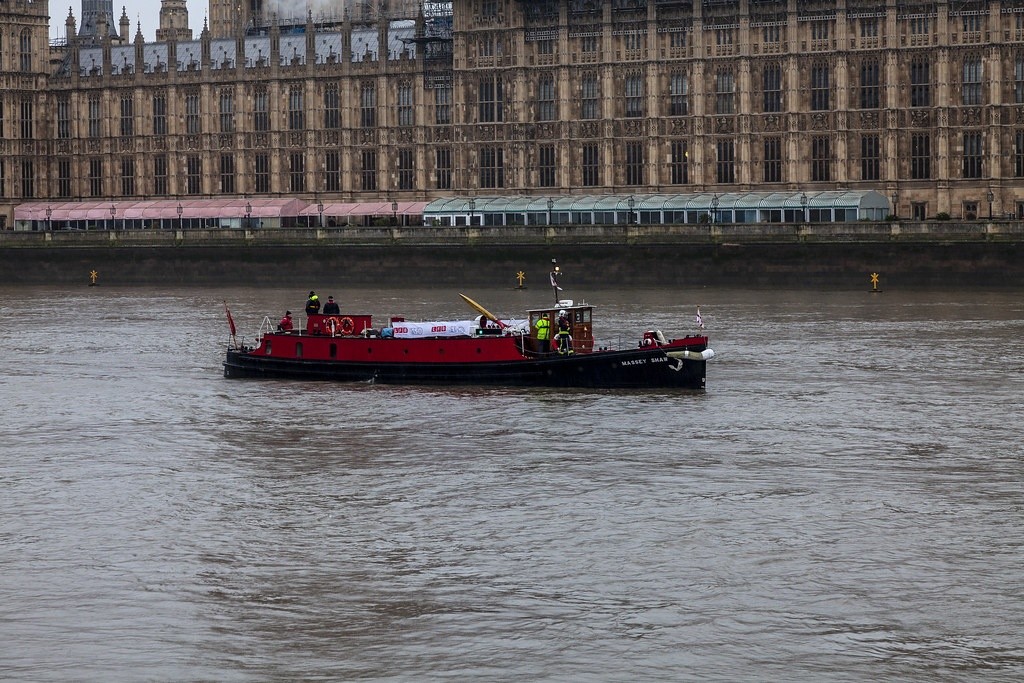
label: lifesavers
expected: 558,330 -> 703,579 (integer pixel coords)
554,333 -> 575,352
340,318 -> 354,335
326,317 -> 339,334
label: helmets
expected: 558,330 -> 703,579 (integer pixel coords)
312,295 -> 318,301
543,314 -> 548,317
558,310 -> 568,318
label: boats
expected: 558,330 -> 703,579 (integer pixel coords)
220,256 -> 715,386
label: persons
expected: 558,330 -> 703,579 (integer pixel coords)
279,310 -> 294,330
323,296 -> 340,315
305,290 -> 321,316
533,310 -> 574,357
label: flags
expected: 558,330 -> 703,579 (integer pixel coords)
224,303 -> 236,336
696,309 -> 705,329
550,273 -> 562,293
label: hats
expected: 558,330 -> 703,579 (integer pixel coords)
286,311 -> 291,316
